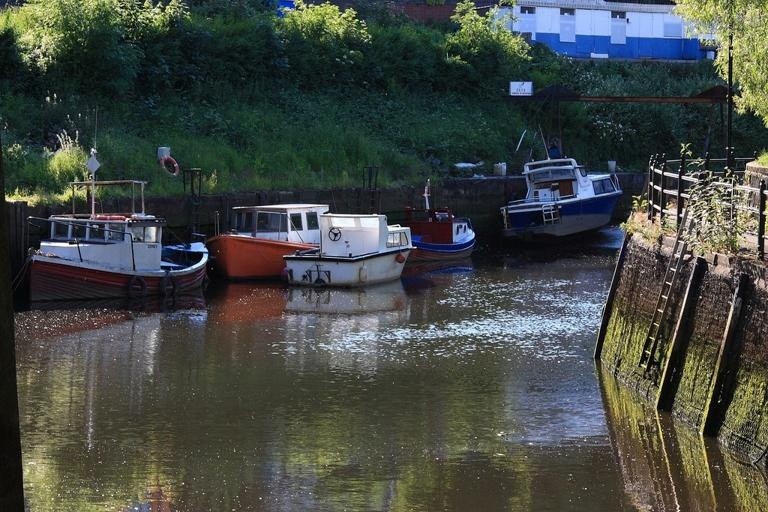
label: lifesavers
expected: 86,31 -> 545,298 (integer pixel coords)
160,156 -> 179,178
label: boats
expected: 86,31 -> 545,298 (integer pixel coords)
29,111 -> 209,304
404,177 -> 478,262
282,158 -> 418,291
206,203 -> 335,283
499,124 -> 620,239
